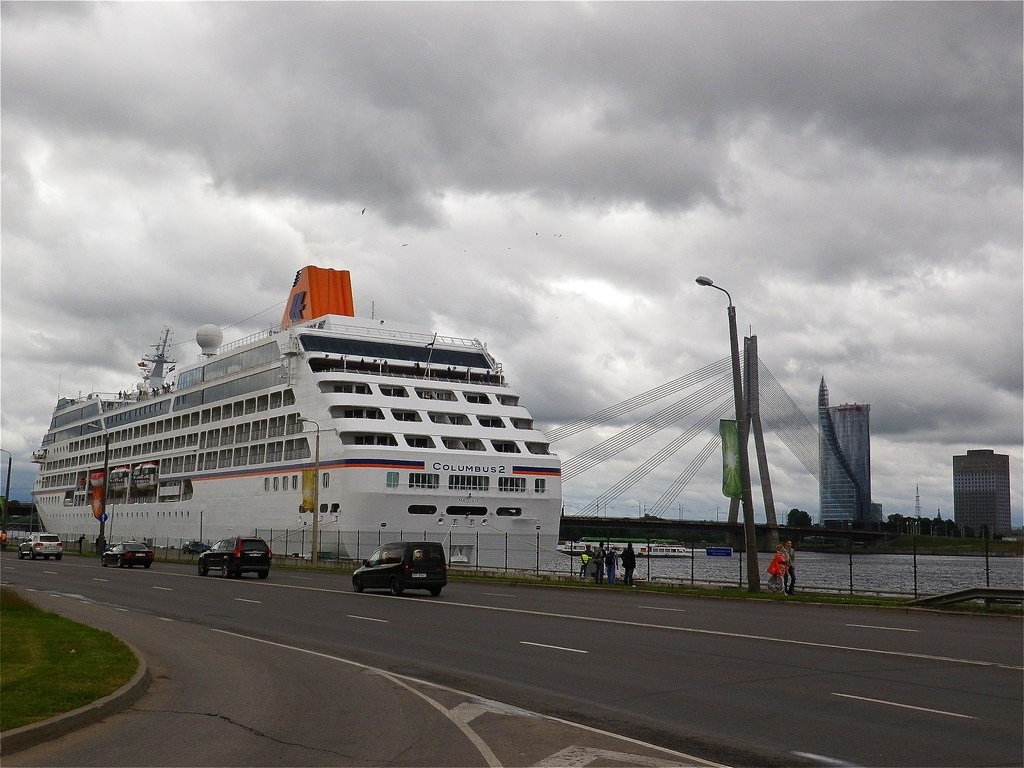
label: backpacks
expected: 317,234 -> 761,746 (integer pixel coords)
605,551 -> 617,566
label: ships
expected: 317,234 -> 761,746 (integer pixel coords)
29,265 -> 562,569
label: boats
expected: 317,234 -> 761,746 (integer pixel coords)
557,540 -> 694,558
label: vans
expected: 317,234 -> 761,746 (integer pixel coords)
351,541 -> 448,597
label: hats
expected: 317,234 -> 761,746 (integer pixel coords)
776,544 -> 784,549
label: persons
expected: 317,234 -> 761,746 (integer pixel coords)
767,544 -> 789,595
78,534 -> 86,553
604,547 -> 620,585
95,535 -> 100,554
103,536 -> 107,552
137,381 -> 174,402
593,542 -> 607,585
118,390 -> 128,400
781,540 -> 798,595
620,543 -> 636,585
0,529 -> 7,549
580,551 -> 589,578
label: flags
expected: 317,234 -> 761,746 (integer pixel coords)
719,419 -> 743,501
92,486 -> 103,521
301,470 -> 316,511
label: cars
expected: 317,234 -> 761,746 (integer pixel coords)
181,539 -> 212,555
101,542 -> 155,569
305,551 -> 339,563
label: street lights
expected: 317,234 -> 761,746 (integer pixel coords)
0,449 -> 12,552
695,275 -> 761,594
88,422 -> 109,556
296,416 -> 320,568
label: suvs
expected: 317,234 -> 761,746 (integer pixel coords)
198,533 -> 273,579
17,533 -> 63,561
